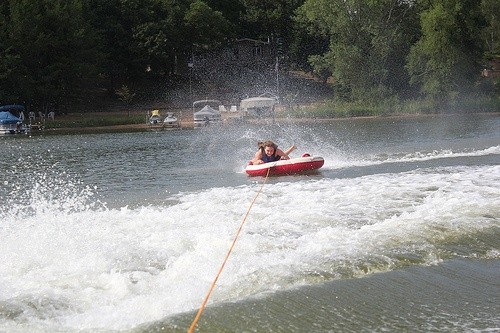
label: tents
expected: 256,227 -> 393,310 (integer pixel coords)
193,105 -> 222,123
0,110 -> 25,130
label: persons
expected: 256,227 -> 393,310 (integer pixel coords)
251,142 -> 297,167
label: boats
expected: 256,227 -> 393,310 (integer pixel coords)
246,154 -> 323,176
